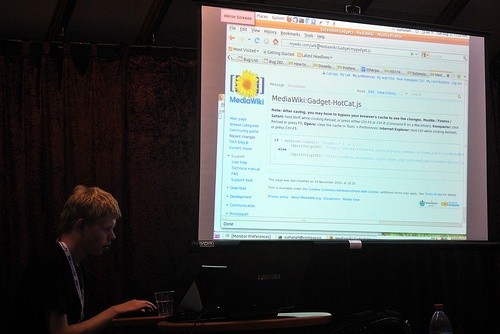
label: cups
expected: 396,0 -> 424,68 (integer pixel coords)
154,290 -> 175,316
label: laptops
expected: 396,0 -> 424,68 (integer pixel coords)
141,265 -> 227,316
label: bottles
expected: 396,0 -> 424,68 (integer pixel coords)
428,303 -> 454,334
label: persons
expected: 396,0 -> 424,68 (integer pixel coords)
0,185 -> 157,334
